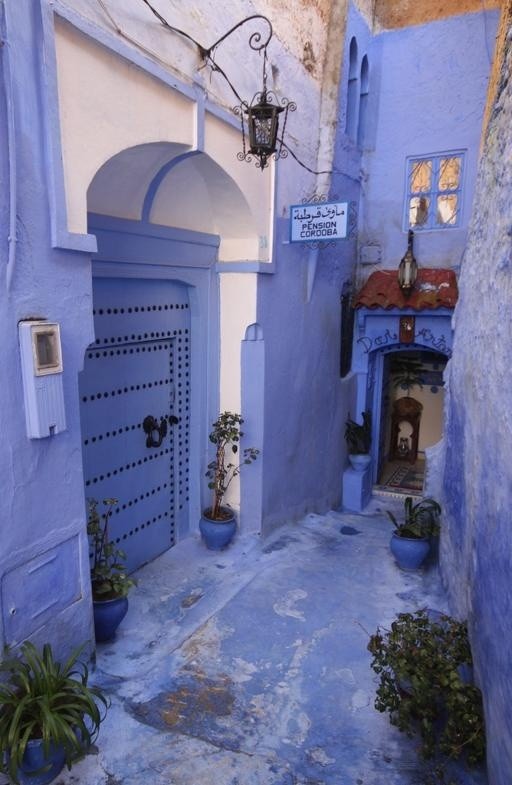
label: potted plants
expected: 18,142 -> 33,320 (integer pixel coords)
87,496 -> 144,644
365,605 -> 486,785
386,497 -> 441,572
0,640 -> 112,785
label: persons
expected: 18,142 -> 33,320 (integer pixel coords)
415,199 -> 426,224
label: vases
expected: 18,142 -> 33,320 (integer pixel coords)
199,410 -> 260,550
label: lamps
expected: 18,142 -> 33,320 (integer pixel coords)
398,229 -> 418,301
232,44 -> 297,172
